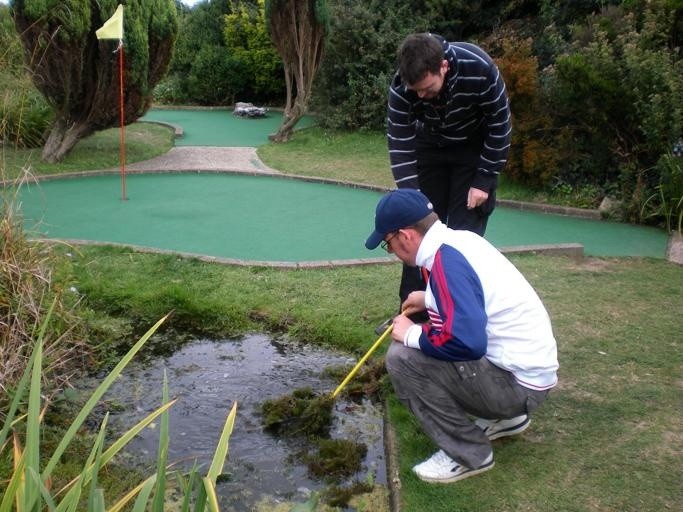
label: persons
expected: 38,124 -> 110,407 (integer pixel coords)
373,31 -> 515,339
362,186 -> 560,483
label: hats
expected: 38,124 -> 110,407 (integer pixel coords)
364,188 -> 434,250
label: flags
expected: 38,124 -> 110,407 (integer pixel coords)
94,4 -> 124,41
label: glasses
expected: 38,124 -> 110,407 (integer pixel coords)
407,85 -> 433,94
380,231 -> 398,251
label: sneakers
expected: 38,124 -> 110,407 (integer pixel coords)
472,413 -> 532,441
412,448 -> 496,484
